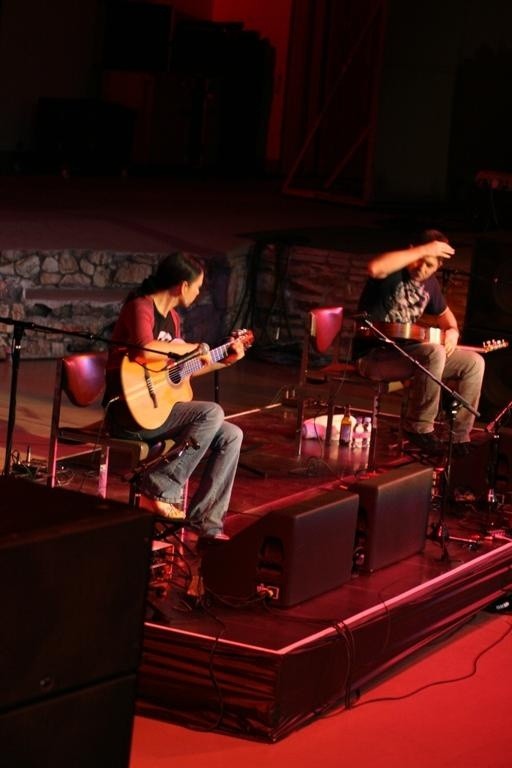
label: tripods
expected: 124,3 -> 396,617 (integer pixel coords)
362,319 -> 481,559
198,370 -> 268,478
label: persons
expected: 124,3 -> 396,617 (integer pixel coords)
101,255 -> 245,546
349,228 -> 485,458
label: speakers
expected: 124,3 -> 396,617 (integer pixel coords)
347,462 -> 434,575
256,489 -> 360,608
0,474 -> 156,768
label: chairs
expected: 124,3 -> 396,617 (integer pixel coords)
47,350 -> 177,509
294,305 -> 415,461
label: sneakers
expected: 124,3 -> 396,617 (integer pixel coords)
400,426 -> 443,456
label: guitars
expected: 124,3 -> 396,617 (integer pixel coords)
108,328 -> 254,431
352,319 -> 508,353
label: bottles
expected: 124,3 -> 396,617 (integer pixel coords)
339,403 -> 352,445
351,417 -> 372,448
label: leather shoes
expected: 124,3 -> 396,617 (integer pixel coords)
204,529 -> 229,542
140,496 -> 186,519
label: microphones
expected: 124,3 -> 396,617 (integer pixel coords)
164,342 -> 210,371
352,311 -> 373,320
